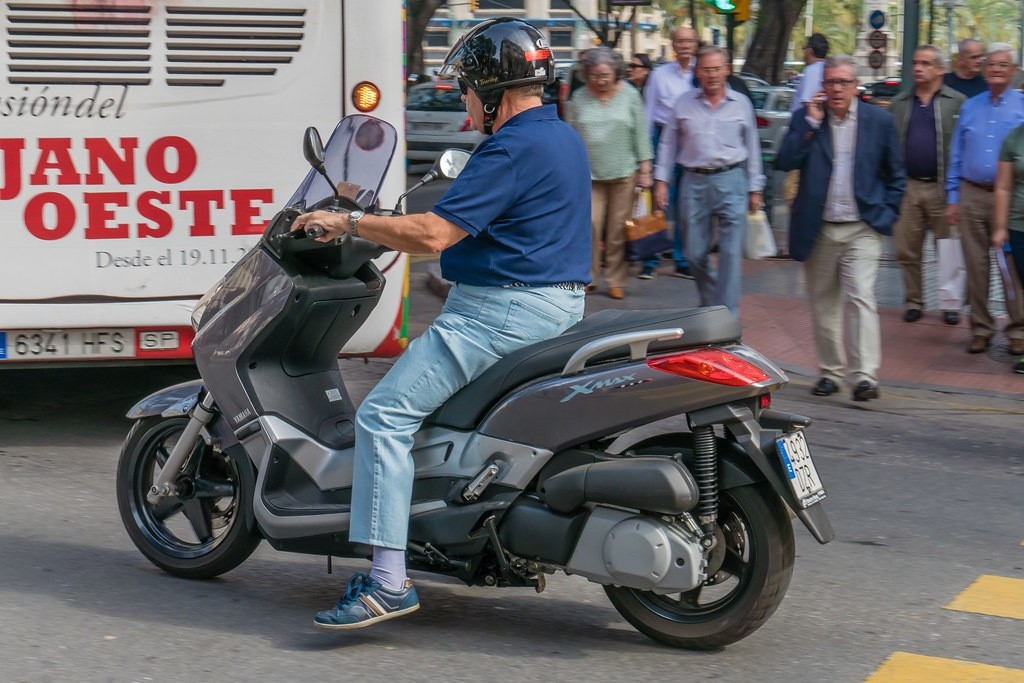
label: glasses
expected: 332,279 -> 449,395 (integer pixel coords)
802,45 -> 811,52
585,70 -> 616,82
961,53 -> 987,62
986,59 -> 1009,69
822,77 -> 854,86
696,66 -> 728,76
455,76 -> 472,95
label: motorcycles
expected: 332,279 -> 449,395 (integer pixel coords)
104,113 -> 839,652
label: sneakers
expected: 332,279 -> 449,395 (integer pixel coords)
904,309 -> 922,322
812,378 -> 838,396
1010,338 -> 1024,354
945,311 -> 960,325
853,380 -> 878,401
967,334 -> 989,353
314,572 -> 421,631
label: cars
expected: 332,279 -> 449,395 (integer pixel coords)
539,64 -> 583,109
407,80 -> 469,112
748,86 -> 801,151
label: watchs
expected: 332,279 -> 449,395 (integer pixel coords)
348,210 -> 364,238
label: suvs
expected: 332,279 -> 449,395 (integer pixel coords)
734,72 -> 774,89
863,77 -> 905,105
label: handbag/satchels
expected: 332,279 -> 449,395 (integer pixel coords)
985,243 -> 1023,331
936,224 -> 970,312
624,209 -> 672,262
632,183 -> 652,218
743,207 -> 776,260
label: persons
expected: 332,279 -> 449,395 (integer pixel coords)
946,42 -> 1024,355
569,51 -> 588,94
483,67 -> 513,82
889,46 -> 967,325
654,47 -> 768,308
290,17 -> 593,629
766,33 -> 829,259
568,46 -> 657,299
773,55 -> 906,401
627,26 -> 750,279
992,120 -> 1024,375
943,38 -> 990,97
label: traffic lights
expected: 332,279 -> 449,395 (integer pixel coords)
716,0 -> 738,14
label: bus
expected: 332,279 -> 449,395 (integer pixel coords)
0,1 -> 411,384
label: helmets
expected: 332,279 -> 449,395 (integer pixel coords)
437,16 -> 555,94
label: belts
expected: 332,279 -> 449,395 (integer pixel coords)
823,220 -> 864,224
909,175 -> 938,183
961,177 -> 995,192
683,159 -> 740,175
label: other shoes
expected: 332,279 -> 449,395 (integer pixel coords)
674,266 -> 696,278
638,266 -> 657,279
609,286 -> 625,299
1013,358 -> 1024,373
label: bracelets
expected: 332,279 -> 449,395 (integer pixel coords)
640,168 -> 651,174
754,193 -> 763,196
